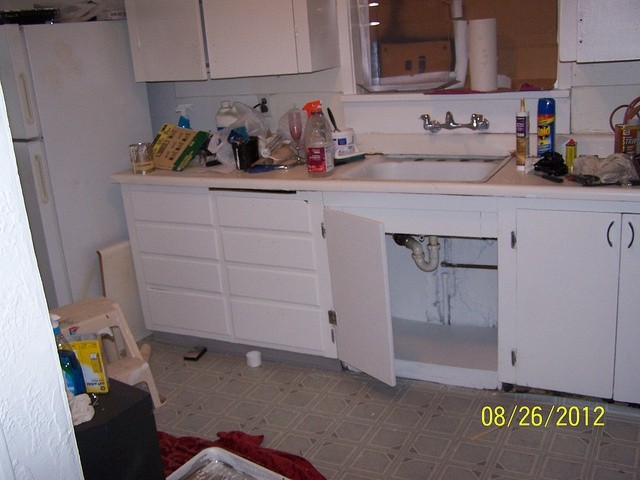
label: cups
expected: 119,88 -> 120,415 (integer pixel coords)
129,143 -> 155,175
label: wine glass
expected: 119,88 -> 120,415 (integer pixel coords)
288,109 -> 304,166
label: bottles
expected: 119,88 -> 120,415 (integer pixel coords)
537,98 -> 556,157
306,107 -> 333,176
517,98 -> 530,170
566,138 -> 577,175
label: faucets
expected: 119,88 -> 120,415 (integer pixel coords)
421,111 -> 490,133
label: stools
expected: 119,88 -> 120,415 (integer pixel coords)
60,294 -> 145,361
102,352 -> 162,410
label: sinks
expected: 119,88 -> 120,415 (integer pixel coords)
341,158 -> 498,182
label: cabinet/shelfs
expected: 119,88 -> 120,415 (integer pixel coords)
127,184 -> 331,363
514,197 -> 640,411
330,191 -> 498,393
124,1 -> 342,85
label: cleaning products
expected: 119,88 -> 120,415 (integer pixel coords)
48,313 -> 87,396
